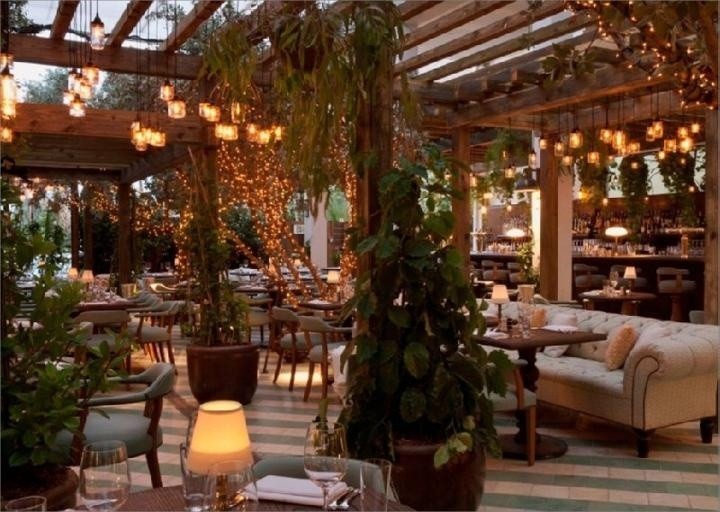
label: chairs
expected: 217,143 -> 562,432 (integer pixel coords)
481,260 -> 510,282
64,360 -> 174,491
126,290 -> 180,381
573,263 -> 606,292
252,456 -> 396,501
237,293 -> 273,348
603,264 -> 647,292
507,262 -> 527,283
656,267 -> 696,323
478,358 -> 537,468
298,315 -> 358,404
272,305 -> 325,394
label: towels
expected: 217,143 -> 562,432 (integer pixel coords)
242,474 -> 347,506
490,333 -> 508,340
542,325 -> 578,333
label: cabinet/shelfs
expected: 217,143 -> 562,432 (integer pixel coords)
492,214 -> 706,249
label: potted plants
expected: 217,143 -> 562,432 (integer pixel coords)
340,132 -> 486,511
182,183 -> 261,407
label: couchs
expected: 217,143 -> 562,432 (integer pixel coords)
475,299 -> 720,459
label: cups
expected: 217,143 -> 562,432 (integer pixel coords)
176,441 -> 215,512
359,458 -> 391,511
204,460 -> 260,512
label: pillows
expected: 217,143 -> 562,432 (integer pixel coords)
530,307 -> 544,325
544,309 -> 577,358
606,323 -> 637,371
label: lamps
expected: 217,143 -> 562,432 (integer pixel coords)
562,129 -> 573,167
623,266 -> 637,296
130,0 -> 186,152
600,100 -> 613,144
627,99 -> 640,152
527,148 -> 537,169
187,400 -> 253,510
664,87 -> 676,153
0,8 -> 17,143
61,0 -> 106,118
81,270 -> 94,291
679,104 -> 689,140
537,110 -> 547,150
505,117 -> 518,179
198,1 -> 287,143
646,90 -> 655,141
68,268 -> 77,280
491,284 -> 509,323
325,271 -> 339,285
587,101 -> 600,165
553,108 -> 565,157
612,100 -> 625,150
570,104 -> 580,149
655,86 -> 664,139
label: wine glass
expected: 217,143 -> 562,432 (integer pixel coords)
305,420 -> 350,512
4,495 -> 48,512
78,438 -> 132,512
